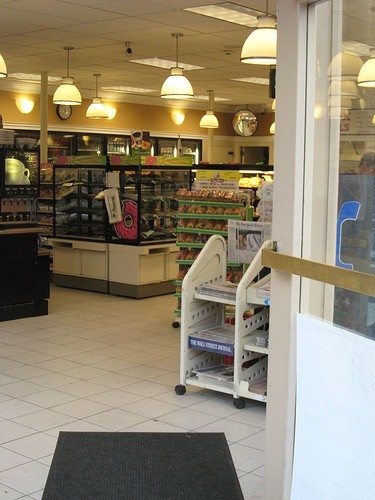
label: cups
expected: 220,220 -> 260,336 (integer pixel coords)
5,158 -> 30,185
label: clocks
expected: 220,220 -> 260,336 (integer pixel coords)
86,74 -> 109,119
56,105 -> 72,120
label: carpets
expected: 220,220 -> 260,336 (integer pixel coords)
42,432 -> 244,500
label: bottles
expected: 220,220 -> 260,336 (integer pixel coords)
1,132 -> 40,150
191,155 -> 195,165
158,144 -> 193,158
48,134 -> 105,163
108,141 -> 125,152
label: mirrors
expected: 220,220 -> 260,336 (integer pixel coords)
231,109 -> 259,136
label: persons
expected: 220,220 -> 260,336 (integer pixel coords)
339,151 -> 375,234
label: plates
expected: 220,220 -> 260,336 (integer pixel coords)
22,175 -> 30,186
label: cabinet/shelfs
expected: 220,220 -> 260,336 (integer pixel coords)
0,145 -> 274,409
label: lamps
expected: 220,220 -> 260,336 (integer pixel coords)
161,33 -> 194,99
240,0 -> 277,65
326,52 -> 364,120
0,55 -> 8,78
199,90 -> 219,129
356,6 -> 375,88
53,47 -> 83,106
269,99 -> 276,135
